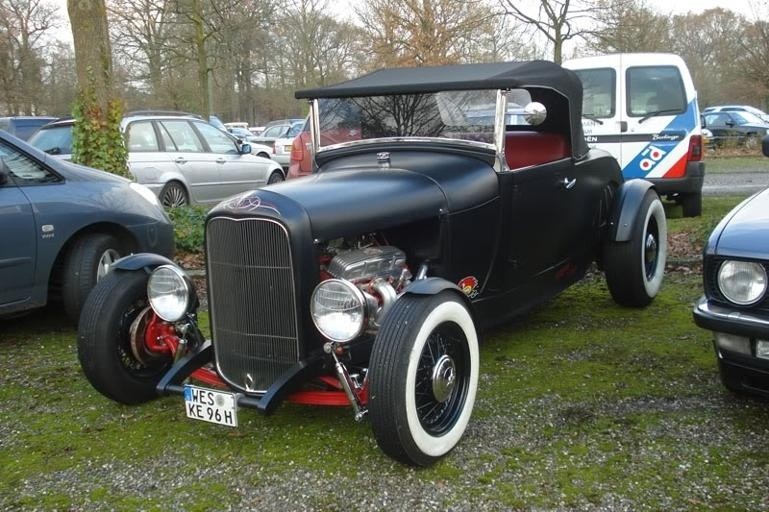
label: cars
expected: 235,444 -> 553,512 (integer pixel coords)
76,59 -> 667,467
692,137 -> 768,400
697,103 -> 767,149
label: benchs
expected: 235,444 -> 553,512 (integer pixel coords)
432,129 -> 568,171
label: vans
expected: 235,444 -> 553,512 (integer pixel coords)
562,52 -> 705,215
